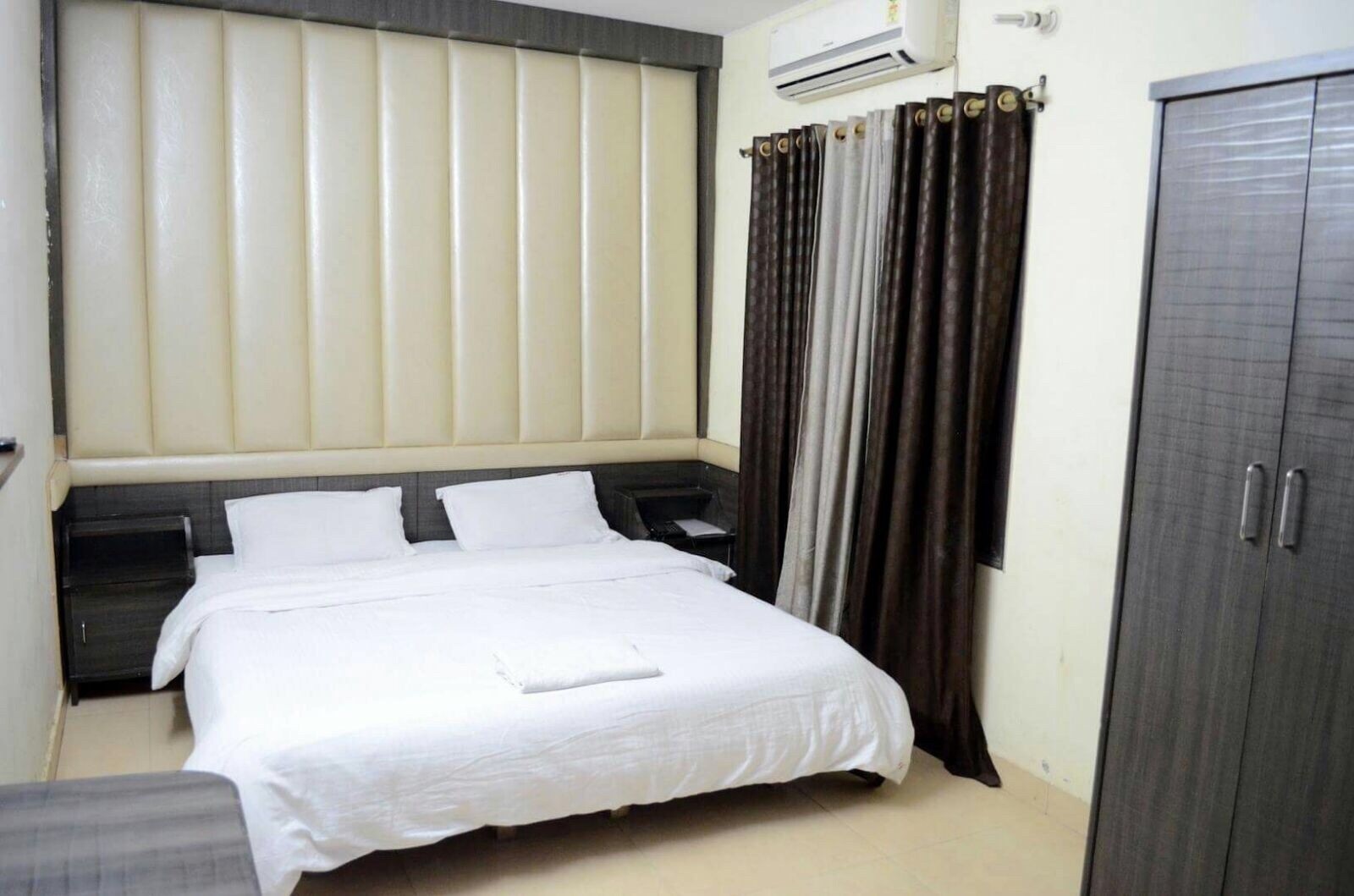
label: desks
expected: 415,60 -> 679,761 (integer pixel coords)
2,771 -> 261,896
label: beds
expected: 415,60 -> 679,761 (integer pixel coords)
150,527 -> 916,896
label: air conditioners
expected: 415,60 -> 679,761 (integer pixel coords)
758,4 -> 962,110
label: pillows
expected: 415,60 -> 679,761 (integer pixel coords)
223,486 -> 418,584
435,471 -> 616,554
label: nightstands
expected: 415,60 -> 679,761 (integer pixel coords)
62,514 -> 201,709
612,482 -> 739,568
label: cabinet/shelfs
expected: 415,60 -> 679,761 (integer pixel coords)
1074,45 -> 1352,896
